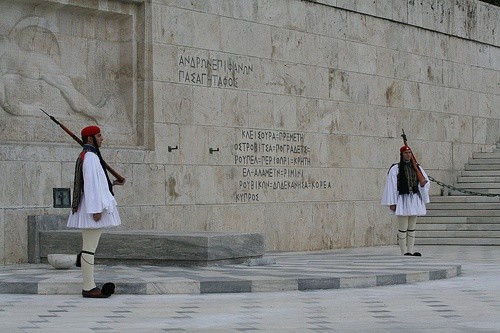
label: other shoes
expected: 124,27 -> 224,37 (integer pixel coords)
403,252 -> 411,255
83,282 -> 115,298
412,252 -> 422,256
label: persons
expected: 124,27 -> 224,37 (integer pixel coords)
66,125 -> 121,299
382,145 -> 431,260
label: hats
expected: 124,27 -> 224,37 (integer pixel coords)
81,126 -> 100,137
400,146 -> 411,151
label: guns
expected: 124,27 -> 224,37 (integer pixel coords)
39,108 -> 125,182
401,128 -> 429,187
